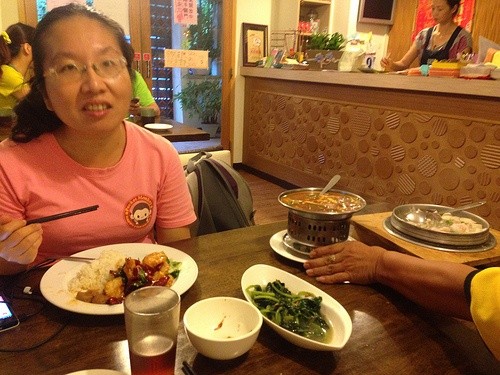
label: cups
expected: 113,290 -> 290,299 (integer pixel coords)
0,108 -> 17,142
122,284 -> 181,375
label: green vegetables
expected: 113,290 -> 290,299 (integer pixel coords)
290,193 -> 338,209
245,279 -> 330,339
122,258 -> 181,284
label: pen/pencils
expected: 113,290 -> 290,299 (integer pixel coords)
387,50 -> 392,62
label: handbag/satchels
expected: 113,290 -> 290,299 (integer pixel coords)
338,41 -> 376,72
351,32 -> 389,72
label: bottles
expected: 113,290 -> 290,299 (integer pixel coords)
129,105 -> 143,127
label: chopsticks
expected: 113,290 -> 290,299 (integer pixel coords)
27,204 -> 99,225
181,360 -> 197,375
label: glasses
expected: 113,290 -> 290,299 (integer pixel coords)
38,55 -> 128,87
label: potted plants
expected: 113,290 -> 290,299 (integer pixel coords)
173,79 -> 222,139
305,29 -> 346,72
181,0 -> 221,75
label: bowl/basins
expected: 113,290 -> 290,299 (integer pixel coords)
142,115 -> 156,126
277,186 -> 368,220
66,369 -> 125,375
241,262 -> 353,352
183,297 -> 263,360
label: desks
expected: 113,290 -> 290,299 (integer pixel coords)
0,199 -> 500,375
0,115 -> 211,143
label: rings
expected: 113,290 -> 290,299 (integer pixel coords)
330,255 -> 336,264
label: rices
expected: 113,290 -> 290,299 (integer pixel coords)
68,250 -> 124,293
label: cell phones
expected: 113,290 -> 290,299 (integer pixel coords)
0,292 -> 20,332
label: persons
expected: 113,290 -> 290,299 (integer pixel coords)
0,22 -> 42,122
0,3 -> 197,279
292,240 -> 500,366
125,37 -> 160,125
380,0 -> 472,72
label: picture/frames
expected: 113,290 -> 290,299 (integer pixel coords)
242,22 -> 269,67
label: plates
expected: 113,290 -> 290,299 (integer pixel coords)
39,243 -> 199,315
143,123 -> 174,131
392,203 -> 490,246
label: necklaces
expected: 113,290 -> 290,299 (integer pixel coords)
431,36 -> 439,48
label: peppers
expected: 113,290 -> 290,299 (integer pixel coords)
107,259 -> 169,306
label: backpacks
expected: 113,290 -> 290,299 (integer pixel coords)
182,152 -> 255,238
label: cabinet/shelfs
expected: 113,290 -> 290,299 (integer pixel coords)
272,0 -> 331,50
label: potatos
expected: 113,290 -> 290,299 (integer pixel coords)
92,294 -> 109,303
103,251 -> 174,296
88,289 -> 99,297
76,291 -> 94,303
